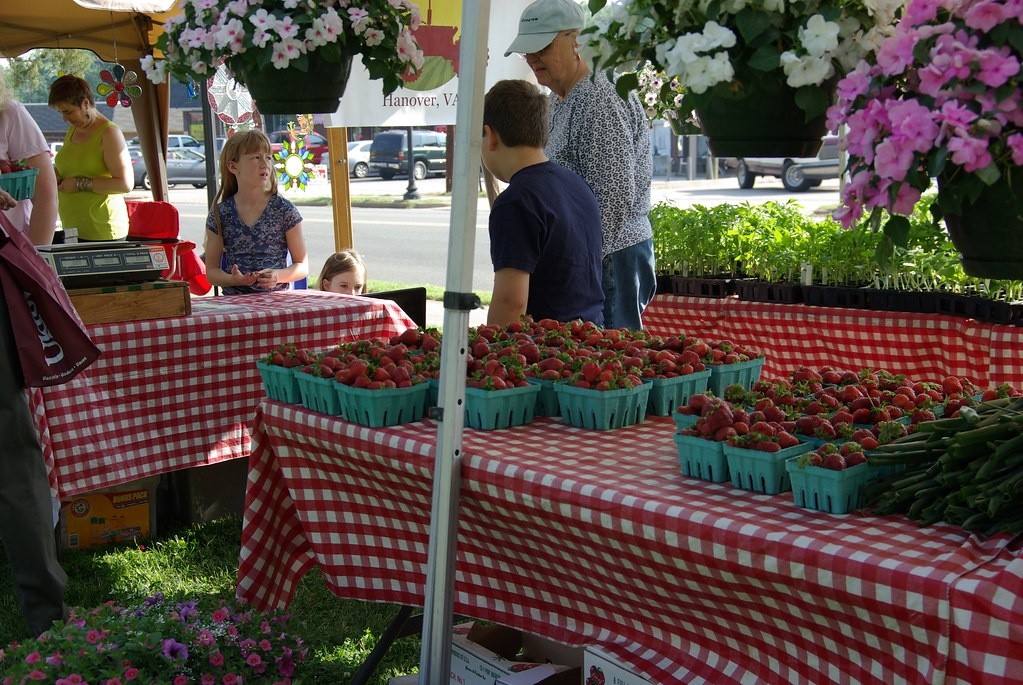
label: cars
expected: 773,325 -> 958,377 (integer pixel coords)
319,139 -> 378,178
133,132 -> 206,154
725,128 -> 841,192
127,146 -> 222,191
216,137 -> 227,160
47,143 -> 64,166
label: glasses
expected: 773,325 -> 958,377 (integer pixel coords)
516,30 -> 575,59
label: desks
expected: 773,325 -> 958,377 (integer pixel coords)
638,293 -> 1022,401
18,287 -> 422,541
233,399 -> 1023,685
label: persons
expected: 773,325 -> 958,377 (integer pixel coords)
48,74 -> 135,242
1,84 -> 58,246
1,185 -> 73,641
206,130 -> 309,296
482,80 -> 605,329
504,0 -> 657,332
315,250 -> 367,296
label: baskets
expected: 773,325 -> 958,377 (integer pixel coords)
252,345 -> 765,430
671,380 -> 984,516
0,168 -> 39,201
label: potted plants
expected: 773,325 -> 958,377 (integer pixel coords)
646,200 -> 1022,330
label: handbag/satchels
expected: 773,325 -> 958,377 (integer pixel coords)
0,210 -> 102,389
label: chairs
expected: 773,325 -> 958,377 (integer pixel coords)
125,200 -> 211,296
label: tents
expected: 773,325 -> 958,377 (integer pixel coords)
1,0 -> 187,202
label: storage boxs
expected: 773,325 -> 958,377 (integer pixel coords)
58,480 -> 154,551
447,620 -> 586,685
582,646 -> 653,685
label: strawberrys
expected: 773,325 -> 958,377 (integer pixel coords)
677,366 -> 1023,470
0,160 -> 32,174
467,315 -> 757,390
273,327 -> 441,389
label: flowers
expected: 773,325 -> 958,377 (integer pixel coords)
573,0 -> 897,137
139,0 -> 425,100
820,0 -> 1022,270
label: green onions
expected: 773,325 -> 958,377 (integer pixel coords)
866,399 -> 1023,534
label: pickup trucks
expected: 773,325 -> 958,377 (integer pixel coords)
268,130 -> 329,164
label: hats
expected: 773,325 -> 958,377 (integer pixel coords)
504,0 -> 586,57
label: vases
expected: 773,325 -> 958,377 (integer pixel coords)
690,58 -> 839,159
238,40 -> 354,113
936,157 -> 1023,281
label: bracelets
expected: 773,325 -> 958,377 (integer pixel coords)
77,176 -> 93,193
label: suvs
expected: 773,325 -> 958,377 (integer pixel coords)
367,130 -> 449,180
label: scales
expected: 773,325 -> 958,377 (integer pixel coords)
34,240 -> 173,289
